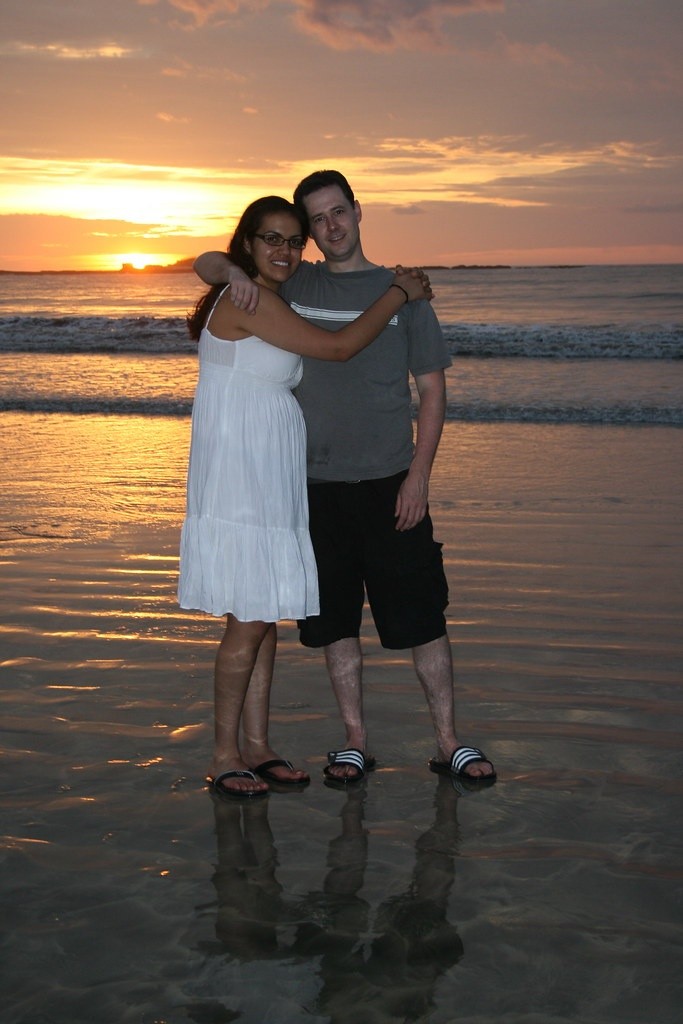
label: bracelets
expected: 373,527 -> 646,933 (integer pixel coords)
390,284 -> 408,304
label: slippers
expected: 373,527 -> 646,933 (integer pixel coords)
324,747 -> 366,782
208,767 -> 268,796
254,759 -> 311,788
430,745 -> 498,785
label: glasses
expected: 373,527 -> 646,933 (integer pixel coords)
254,233 -> 306,249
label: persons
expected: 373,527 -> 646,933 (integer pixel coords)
190,169 -> 499,786
175,196 -> 438,797
